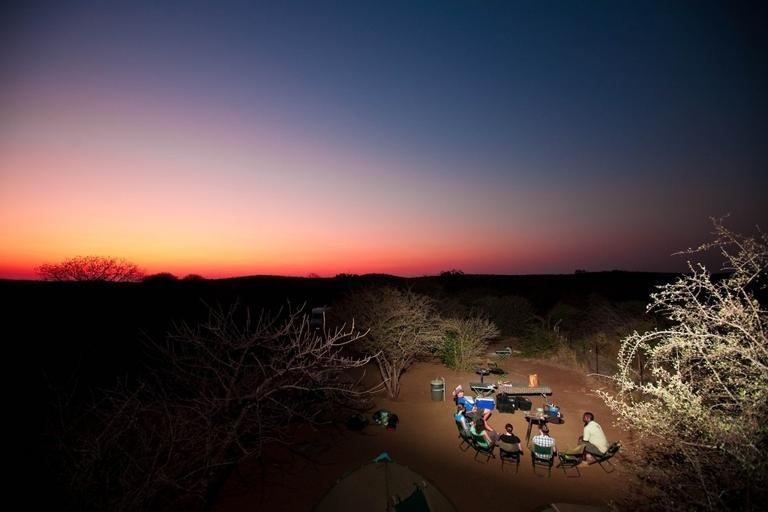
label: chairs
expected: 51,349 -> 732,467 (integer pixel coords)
454,411 -> 620,477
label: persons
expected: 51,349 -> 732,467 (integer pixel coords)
579,412 -> 609,464
455,404 -> 475,431
498,423 -> 523,464
470,419 -> 499,449
530,422 -> 557,466
451,383 -> 494,433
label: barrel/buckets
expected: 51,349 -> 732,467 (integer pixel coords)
430,380 -> 443,400
548,403 -> 561,414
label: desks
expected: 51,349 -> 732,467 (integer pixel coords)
470,383 -> 552,405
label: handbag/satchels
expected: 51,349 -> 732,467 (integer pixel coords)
497,394 -> 532,414
